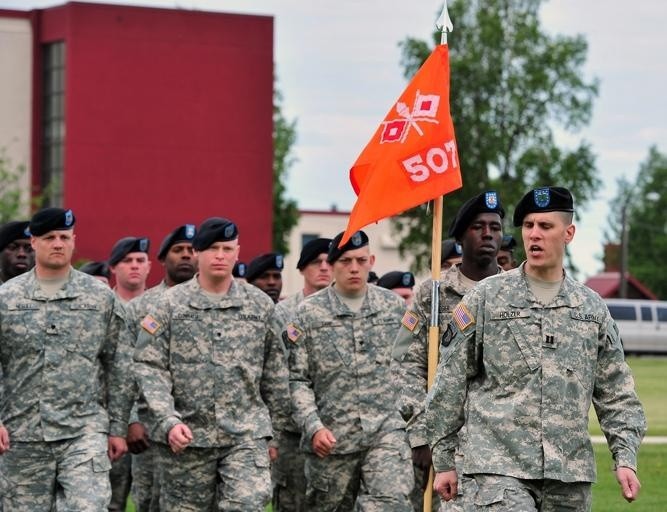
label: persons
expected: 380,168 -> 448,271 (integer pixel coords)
390,190 -> 520,511
420,183 -> 648,511
109,216 -> 287,511
0,206 -> 136,511
272,231 -> 414,511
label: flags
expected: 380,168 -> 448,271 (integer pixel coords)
334,43 -> 464,251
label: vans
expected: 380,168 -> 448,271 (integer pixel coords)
601,296 -> 667,356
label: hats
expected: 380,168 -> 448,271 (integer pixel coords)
428,237 -> 463,269
376,269 -> 416,288
500,235 -> 515,253
327,230 -> 368,264
78,218 -> 283,284
447,190 -> 504,240
297,237 -> 333,269
513,186 -> 573,226
1,208 -> 75,250
